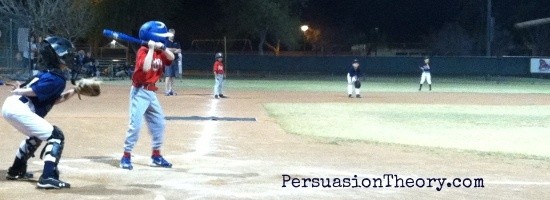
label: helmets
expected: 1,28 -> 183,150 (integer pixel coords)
139,20 -> 173,48
215,52 -> 223,62
40,35 -> 83,84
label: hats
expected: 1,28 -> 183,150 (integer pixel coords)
352,59 -> 359,64
424,56 -> 430,59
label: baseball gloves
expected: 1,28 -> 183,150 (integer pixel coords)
74,78 -> 102,97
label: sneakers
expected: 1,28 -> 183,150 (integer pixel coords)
5,166 -> 33,180
120,157 -> 133,170
37,176 -> 70,189
151,155 -> 172,168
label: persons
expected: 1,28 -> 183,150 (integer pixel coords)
1,35 -> 99,191
213,53 -> 225,99
82,52 -> 97,77
120,21 -> 175,169
347,59 -> 361,98
22,34 -> 37,69
165,28 -> 182,96
418,56 -> 432,91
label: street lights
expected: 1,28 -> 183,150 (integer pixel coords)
300,24 -> 309,55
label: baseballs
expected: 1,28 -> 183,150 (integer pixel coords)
301,25 -> 308,31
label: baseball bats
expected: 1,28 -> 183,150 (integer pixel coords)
103,29 -> 165,51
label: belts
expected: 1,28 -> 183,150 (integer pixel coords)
19,95 -> 29,104
131,81 -> 159,91
